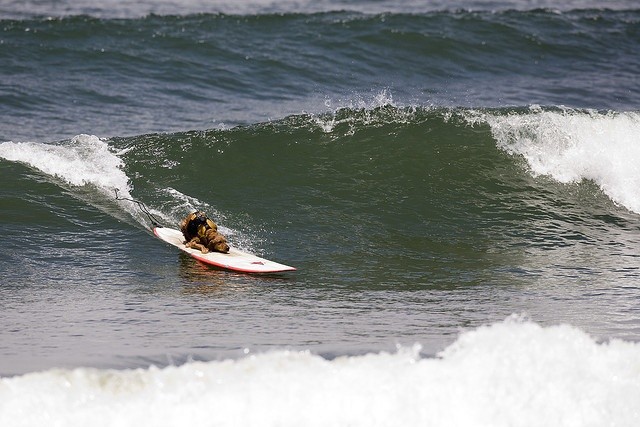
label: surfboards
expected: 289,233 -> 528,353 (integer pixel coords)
154,227 -> 297,273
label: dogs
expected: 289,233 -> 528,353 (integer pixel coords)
178,211 -> 230,254
